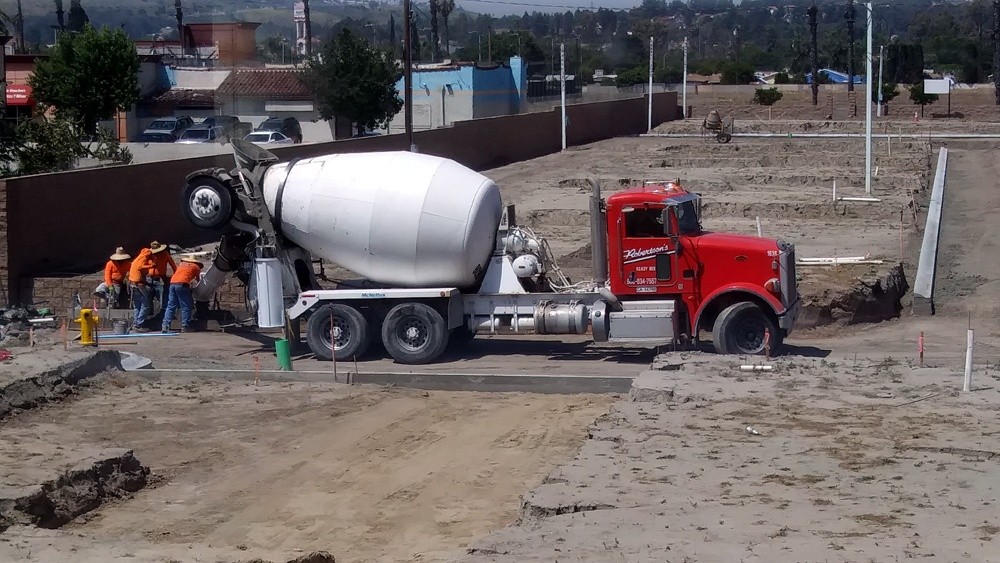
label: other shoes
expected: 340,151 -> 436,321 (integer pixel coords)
181,327 -> 196,333
132,324 -> 150,332
161,326 -> 167,333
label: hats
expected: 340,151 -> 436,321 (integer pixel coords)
150,241 -> 167,254
109,247 -> 132,260
181,255 -> 204,269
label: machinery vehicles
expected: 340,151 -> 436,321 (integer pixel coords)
182,137 -> 803,365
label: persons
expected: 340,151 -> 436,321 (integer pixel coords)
105,246 -> 132,310
161,254 -> 204,334
129,248 -> 158,333
144,240 -> 178,316
914,110 -> 919,121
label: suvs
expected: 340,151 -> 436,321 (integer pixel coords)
253,117 -> 303,144
174,124 -> 229,144
143,115 -> 194,143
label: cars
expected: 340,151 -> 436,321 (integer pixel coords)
241,130 -> 294,146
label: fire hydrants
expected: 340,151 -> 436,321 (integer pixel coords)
73,308 -> 99,346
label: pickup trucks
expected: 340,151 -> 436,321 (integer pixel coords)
201,115 -> 253,143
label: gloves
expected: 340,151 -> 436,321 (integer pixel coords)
108,286 -> 115,294
